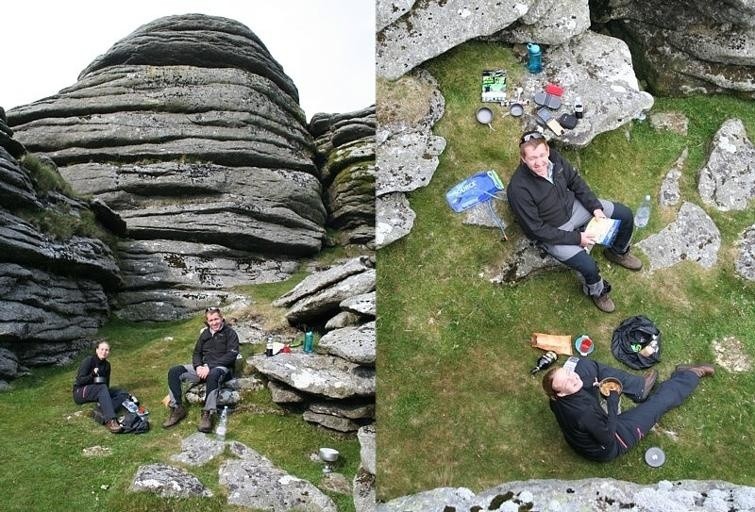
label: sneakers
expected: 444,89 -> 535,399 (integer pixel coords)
602,250 -> 643,271
675,364 -> 715,379
592,294 -> 615,313
106,419 -> 121,433
198,414 -> 212,432
640,369 -> 657,402
162,407 -> 186,429
87,411 -> 104,424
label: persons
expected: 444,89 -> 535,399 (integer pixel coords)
507,131 -> 642,313
542,357 -> 715,462
74,339 -> 128,433
162,307 -> 239,433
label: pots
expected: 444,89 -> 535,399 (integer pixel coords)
476,106 -> 497,132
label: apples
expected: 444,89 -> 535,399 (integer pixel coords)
581,339 -> 592,352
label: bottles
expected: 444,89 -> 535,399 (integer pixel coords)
215,405 -> 227,443
530,350 -> 559,375
573,97 -> 583,118
633,194 -> 652,228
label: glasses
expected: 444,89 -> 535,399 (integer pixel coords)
519,133 -> 546,147
206,307 -> 220,312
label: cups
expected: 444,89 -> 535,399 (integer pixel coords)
304,331 -> 314,354
266,337 -> 273,357
526,42 -> 543,74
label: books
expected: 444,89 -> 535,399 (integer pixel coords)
585,217 -> 622,248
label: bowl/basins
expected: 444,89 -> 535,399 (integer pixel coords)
598,376 -> 624,400
320,447 -> 340,462
510,103 -> 523,118
574,335 -> 595,357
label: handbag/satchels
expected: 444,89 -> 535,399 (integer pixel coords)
612,316 -> 662,369
119,412 -> 149,434
